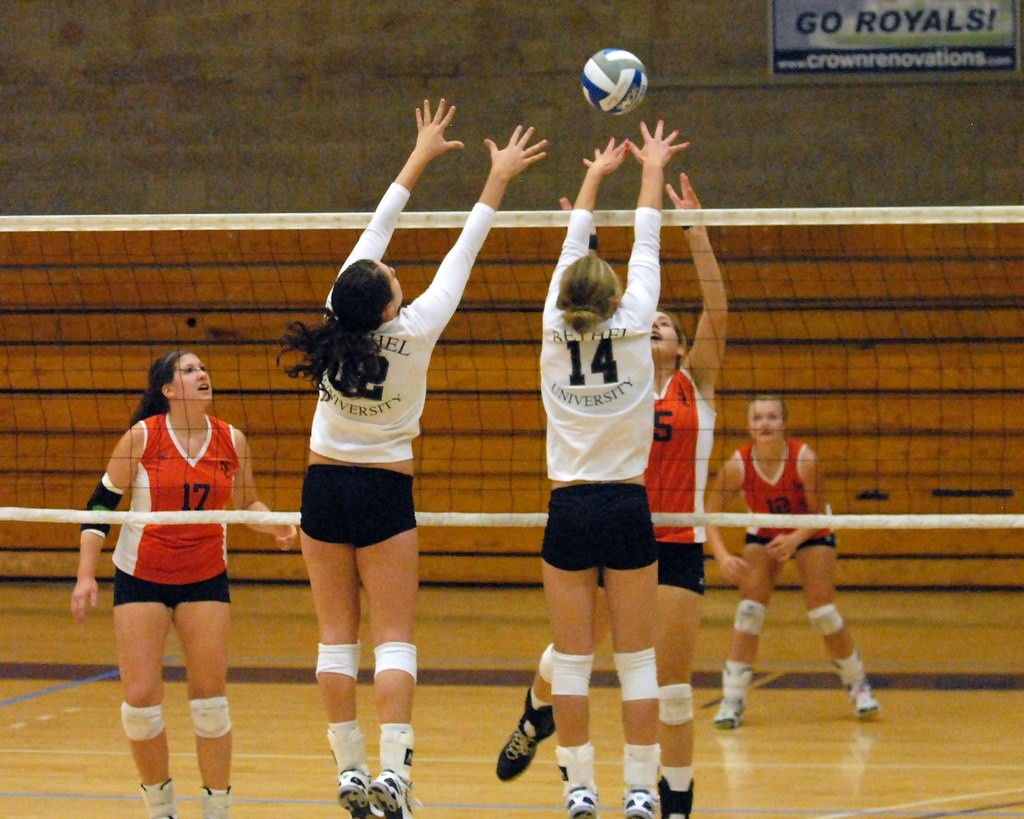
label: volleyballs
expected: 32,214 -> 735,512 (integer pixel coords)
579,45 -> 650,119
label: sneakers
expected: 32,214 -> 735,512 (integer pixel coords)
713,696 -> 745,730
496,687 -> 555,782
847,677 -> 879,717
368,763 -> 422,819
658,775 -> 694,819
337,766 -> 373,819
565,785 -> 598,819
623,785 -> 660,819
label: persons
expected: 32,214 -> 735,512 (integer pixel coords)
493,120 -> 729,819
70,348 -> 303,817
271,95 -> 549,819
703,390 -> 882,728
